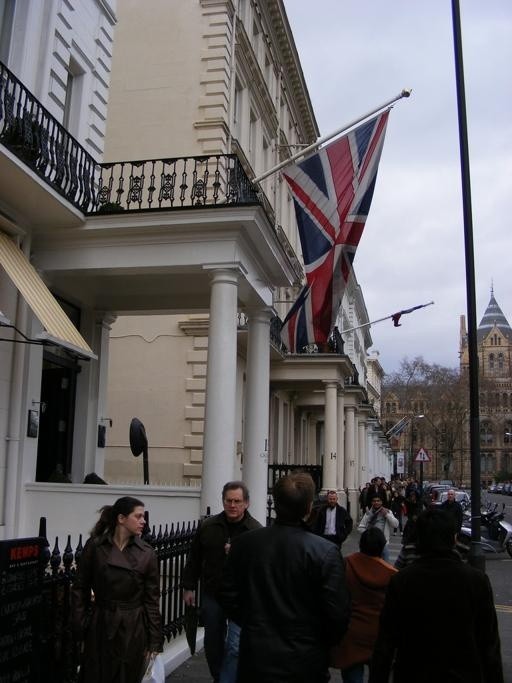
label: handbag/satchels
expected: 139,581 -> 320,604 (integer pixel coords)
141,653 -> 165,683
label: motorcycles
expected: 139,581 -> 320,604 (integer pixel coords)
447,496 -> 512,561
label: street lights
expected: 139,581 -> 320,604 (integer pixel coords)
410,412 -> 424,479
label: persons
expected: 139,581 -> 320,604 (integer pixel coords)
312,490 -> 354,551
358,475 -> 428,536
68,496 -> 173,682
341,526 -> 396,683
368,504 -> 503,682
356,494 -> 400,559
226,471 -> 347,682
394,500 -> 472,570
181,481 -> 258,682
437,488 -> 464,536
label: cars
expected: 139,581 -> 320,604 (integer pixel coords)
487,482 -> 512,495
422,479 -> 470,508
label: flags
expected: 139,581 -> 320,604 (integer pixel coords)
391,304 -> 426,327
279,106 -> 395,354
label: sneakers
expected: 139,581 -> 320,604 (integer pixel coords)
393,532 -> 403,536
